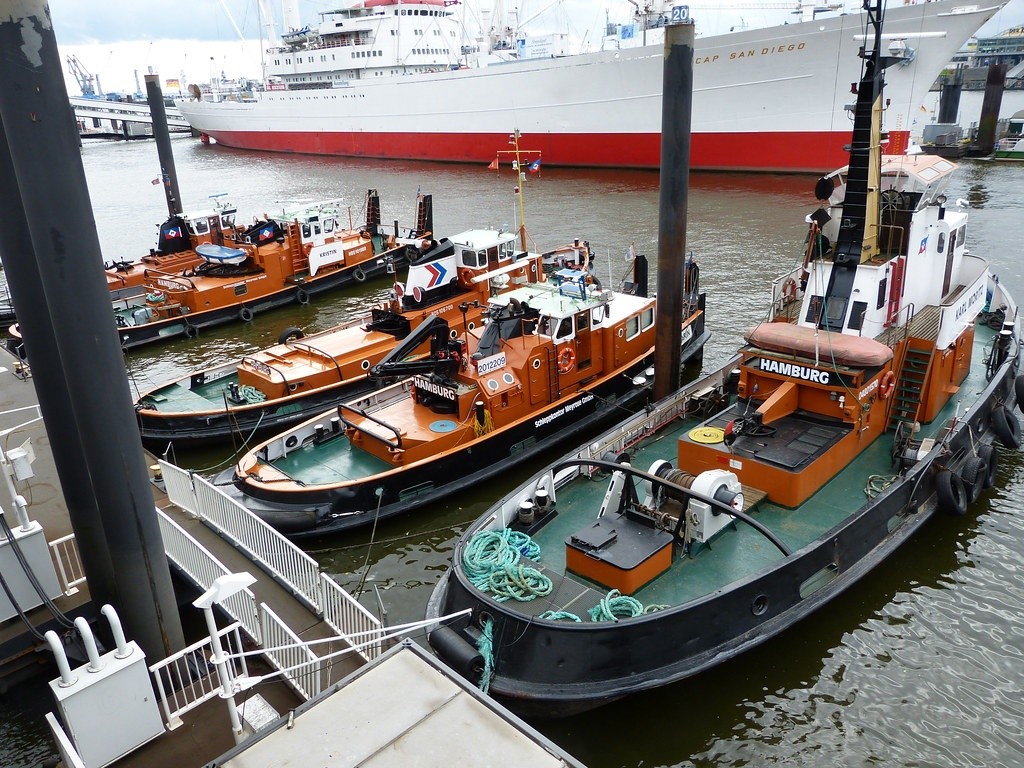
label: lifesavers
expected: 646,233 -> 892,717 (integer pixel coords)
185,324 -> 199,338
557,347 -> 576,372
461,268 -> 475,287
992,403 -> 1022,449
405,249 -> 418,262
1014,373 -> 1024,412
783,278 -> 797,303
962,457 -> 985,503
354,270 -> 367,282
978,444 -> 998,488
879,370 -> 896,399
240,308 -> 253,322
278,327 -> 304,345
938,469 -> 968,514
295,289 -> 310,305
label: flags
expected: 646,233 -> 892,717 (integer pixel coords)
444,0 -> 462,6
529,159 -> 539,175
624,245 -> 636,263
488,157 -> 497,171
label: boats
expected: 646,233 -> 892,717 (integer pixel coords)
173,0 -> 1009,177
0,166 -> 435,352
135,128 -> 711,547
425,0 -> 1024,720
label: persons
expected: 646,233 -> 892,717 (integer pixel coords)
211,219 -> 219,228
